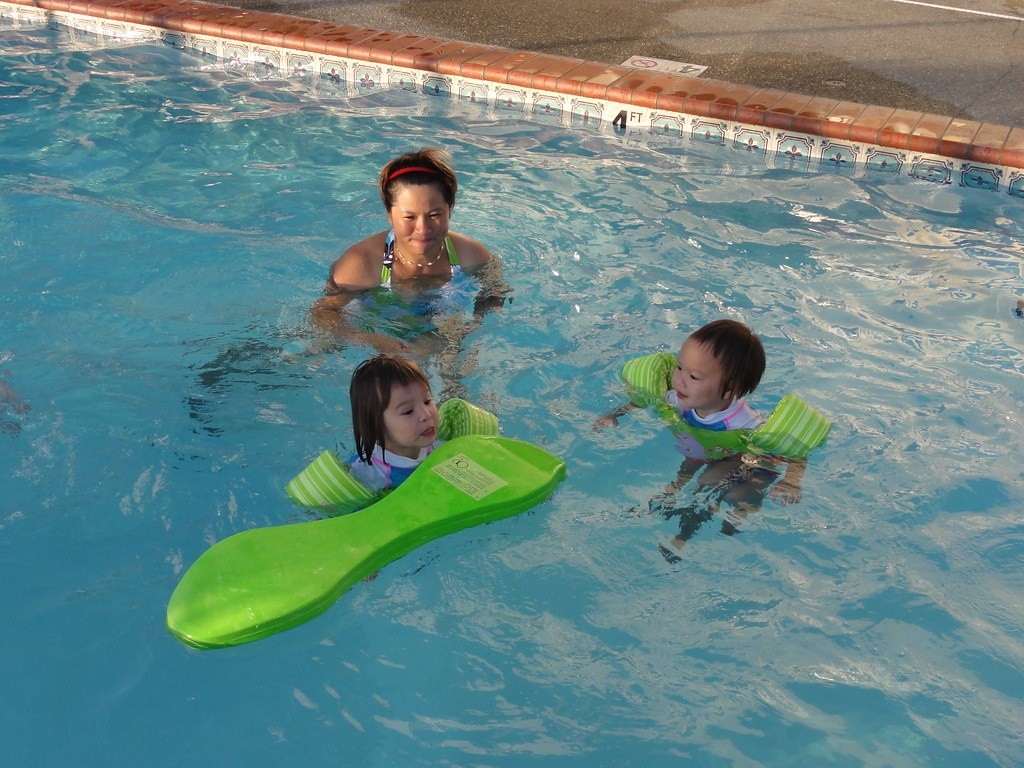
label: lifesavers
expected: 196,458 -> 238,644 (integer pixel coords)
286,451 -> 375,507
622,353 -> 678,406
748,394 -> 832,460
435,399 -> 500,442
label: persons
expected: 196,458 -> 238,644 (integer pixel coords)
349,354 -> 440,469
666,319 -> 765,430
334,148 -> 490,290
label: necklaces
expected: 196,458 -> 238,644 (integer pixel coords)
395,242 -> 444,266
